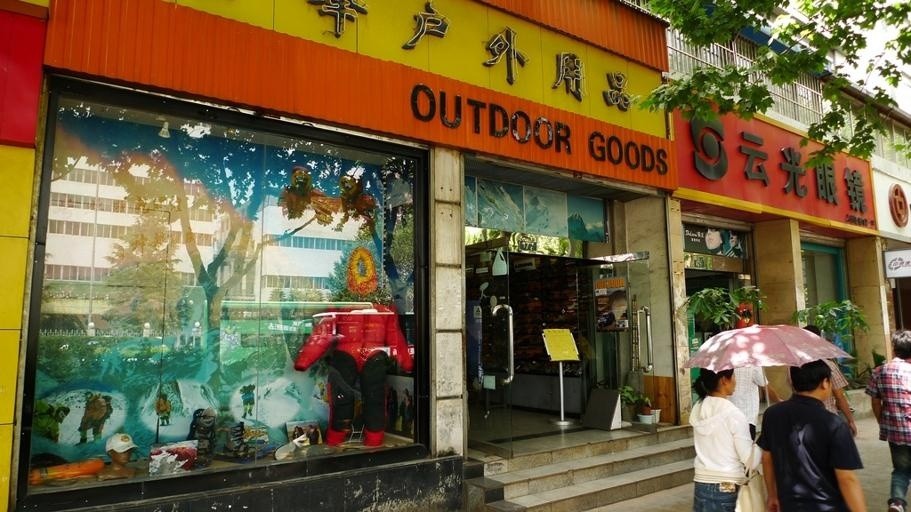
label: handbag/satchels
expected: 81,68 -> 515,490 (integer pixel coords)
732,443 -> 768,512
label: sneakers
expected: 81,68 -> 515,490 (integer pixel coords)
888,503 -> 904,511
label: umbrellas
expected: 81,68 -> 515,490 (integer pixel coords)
684,323 -> 856,408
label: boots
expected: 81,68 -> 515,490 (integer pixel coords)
224,422 -> 250,452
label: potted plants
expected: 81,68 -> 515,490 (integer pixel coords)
615,383 -> 662,425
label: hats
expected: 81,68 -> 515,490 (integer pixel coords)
105,433 -> 137,453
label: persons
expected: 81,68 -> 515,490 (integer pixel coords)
688,364 -> 782,512
756,325 -> 867,512
865,329 -> 911,512
